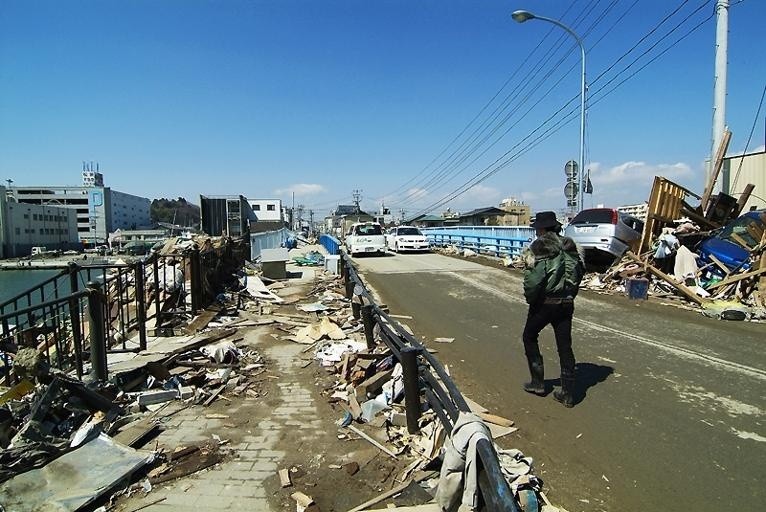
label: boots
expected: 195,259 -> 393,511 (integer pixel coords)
522,355 -> 545,393
552,356 -> 575,408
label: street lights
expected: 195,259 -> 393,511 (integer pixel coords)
511,10 -> 585,212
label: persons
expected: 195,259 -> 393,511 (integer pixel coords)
523,211 -> 586,408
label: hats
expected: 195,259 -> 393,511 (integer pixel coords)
529,211 -> 564,229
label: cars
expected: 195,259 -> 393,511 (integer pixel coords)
343,222 -> 431,257
564,209 -> 644,259
694,211 -> 766,281
